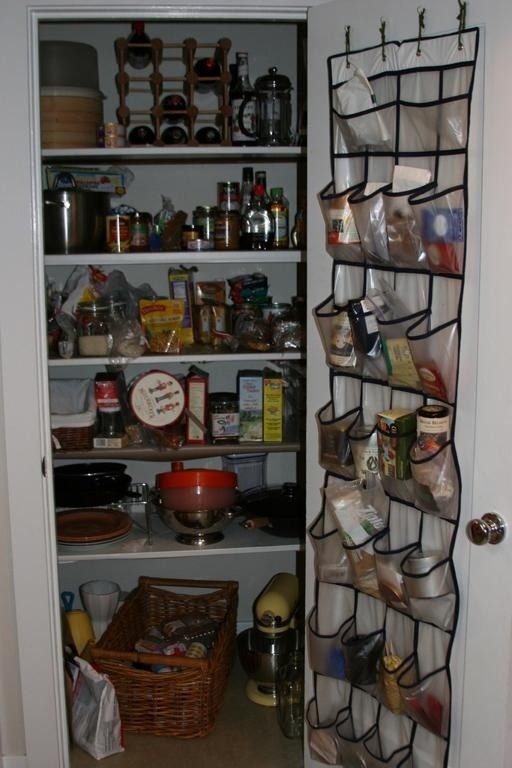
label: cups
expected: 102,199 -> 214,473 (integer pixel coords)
356,423 -> 379,446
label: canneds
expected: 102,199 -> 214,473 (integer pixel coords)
194,206 -> 214,250
129,212 -> 150,251
209,393 -> 241,445
183,224 -> 202,250
218,180 -> 240,212
213,212 -> 239,250
261,303 -> 290,324
105,215 -> 131,254
103,123 -> 126,148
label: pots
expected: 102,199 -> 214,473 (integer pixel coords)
43,170 -> 111,252
54,462 -> 133,506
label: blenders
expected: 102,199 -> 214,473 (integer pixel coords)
237,573 -> 303,706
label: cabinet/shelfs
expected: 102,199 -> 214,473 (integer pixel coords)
32,14 -> 312,767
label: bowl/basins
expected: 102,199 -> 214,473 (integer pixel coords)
148,495 -> 237,546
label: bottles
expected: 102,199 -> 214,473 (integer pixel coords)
270,188 -> 288,248
195,125 -> 220,144
96,372 -> 122,437
194,57 -> 221,89
415,403 -> 450,444
230,52 -> 257,143
161,623 -> 217,648
128,126 -> 154,145
77,302 -> 114,357
244,198 -> 273,248
161,126 -> 187,145
126,21 -> 152,70
240,167 -> 254,217
181,224 -> 204,252
254,171 -> 268,208
159,94 -> 186,124
272,651 -> 304,742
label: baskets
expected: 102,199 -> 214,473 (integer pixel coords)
48,379 -> 94,451
90,576 -> 239,740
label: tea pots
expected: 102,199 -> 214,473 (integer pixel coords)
239,66 -> 292,146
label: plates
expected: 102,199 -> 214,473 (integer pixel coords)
52,507 -> 131,548
